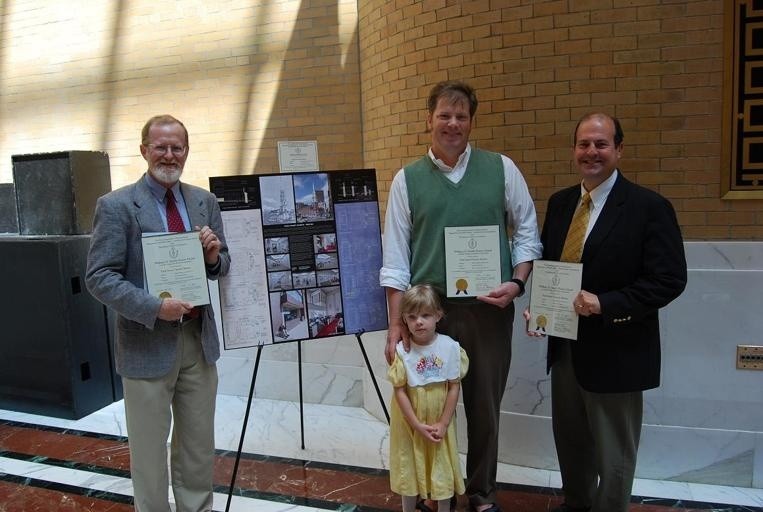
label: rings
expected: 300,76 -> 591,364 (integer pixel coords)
575,304 -> 584,309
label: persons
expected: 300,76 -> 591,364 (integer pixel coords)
385,280 -> 469,511
376,80 -> 543,512
524,112 -> 688,512
83,114 -> 232,511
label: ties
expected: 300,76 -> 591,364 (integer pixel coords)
560,191 -> 591,263
164,187 -> 198,319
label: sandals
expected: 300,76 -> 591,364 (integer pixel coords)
416,494 -> 456,512
467,499 -> 504,511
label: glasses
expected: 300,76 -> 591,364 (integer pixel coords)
146,139 -> 188,156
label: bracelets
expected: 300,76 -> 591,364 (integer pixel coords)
508,276 -> 527,301
